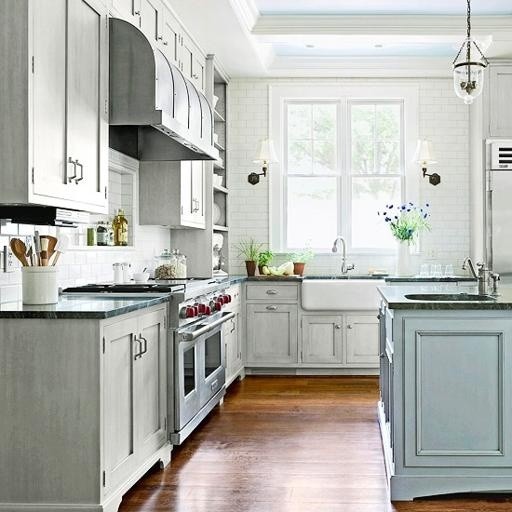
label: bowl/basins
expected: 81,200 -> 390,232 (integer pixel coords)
134,272 -> 150,285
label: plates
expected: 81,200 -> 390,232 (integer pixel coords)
213,202 -> 223,225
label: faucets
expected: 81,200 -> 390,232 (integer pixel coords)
332,236 -> 355,275
462,256 -> 500,296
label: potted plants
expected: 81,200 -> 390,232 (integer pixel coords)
236,236 -> 263,277
257,251 -> 274,275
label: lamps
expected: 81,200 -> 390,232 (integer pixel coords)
246,138 -> 276,187
412,140 -> 440,186
450,0 -> 491,105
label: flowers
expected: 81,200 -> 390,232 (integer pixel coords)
377,201 -> 431,239
286,250 -> 313,262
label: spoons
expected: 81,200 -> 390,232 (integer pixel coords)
10,238 -> 29,265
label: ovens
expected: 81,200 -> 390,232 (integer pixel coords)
177,312 -> 235,433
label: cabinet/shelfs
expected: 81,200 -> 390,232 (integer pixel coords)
140,32 -> 208,230
0,0 -> 112,214
378,296 -> 511,502
243,280 -> 302,377
298,311 -> 378,376
139,0 -> 180,65
110,0 -> 138,30
0,299 -> 173,512
223,282 -> 245,391
171,64 -> 230,278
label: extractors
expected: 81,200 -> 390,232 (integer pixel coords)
109,18 -> 217,160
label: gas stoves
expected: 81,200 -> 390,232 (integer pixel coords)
61,283 -> 186,292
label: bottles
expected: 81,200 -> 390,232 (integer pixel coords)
118,208 -> 128,245
96,220 -> 107,245
114,216 -> 122,246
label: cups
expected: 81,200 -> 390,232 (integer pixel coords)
434,265 -> 443,278
421,265 -> 429,278
446,265 -> 454,278
430,265 -> 436,277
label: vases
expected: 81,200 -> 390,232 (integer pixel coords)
394,237 -> 413,278
293,261 -> 305,275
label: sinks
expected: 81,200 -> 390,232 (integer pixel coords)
300,275 -> 386,311
403,292 -> 498,303
